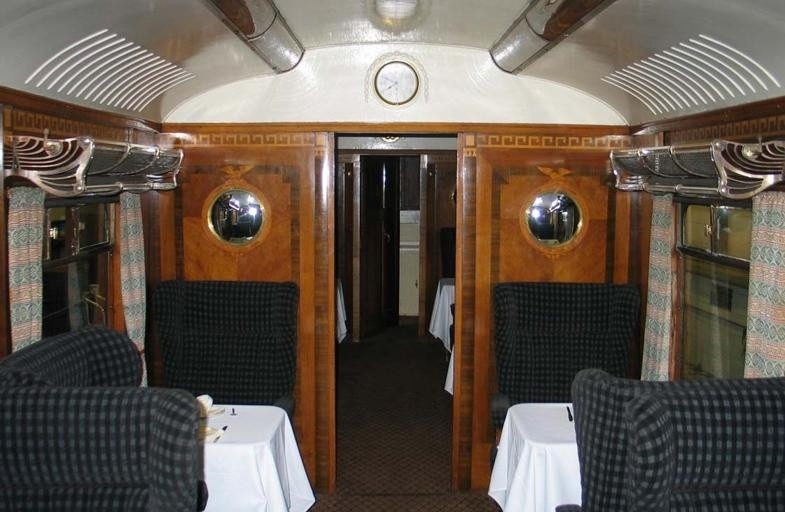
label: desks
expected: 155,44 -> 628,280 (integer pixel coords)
488,402 -> 581,512
201,404 -> 316,511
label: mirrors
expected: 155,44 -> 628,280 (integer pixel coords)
519,165 -> 591,260
198,164 -> 269,253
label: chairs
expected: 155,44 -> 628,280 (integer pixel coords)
570,367 -> 785,512
3,385 -> 208,511
1,322 -> 144,387
496,285 -> 649,403
146,278 -> 296,420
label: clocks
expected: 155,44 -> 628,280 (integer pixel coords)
375,61 -> 417,105
